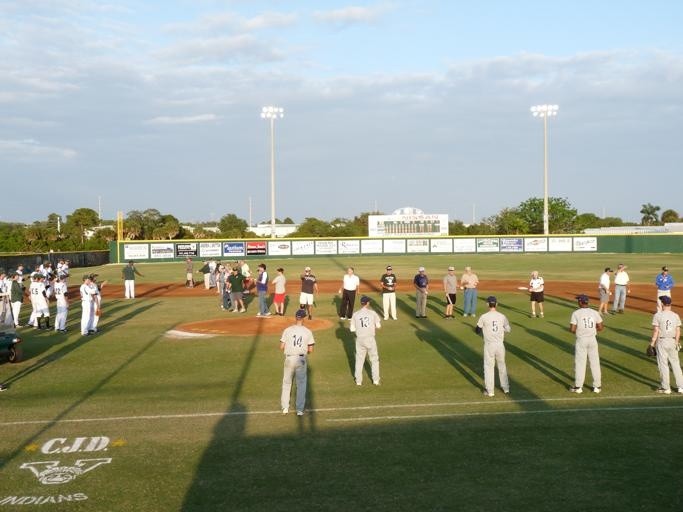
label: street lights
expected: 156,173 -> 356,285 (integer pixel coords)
529,102 -> 557,233
260,104 -> 286,235
98,196 -> 101,226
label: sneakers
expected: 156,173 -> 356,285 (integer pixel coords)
483,390 -> 494,398
656,387 -> 671,394
678,387 -> 683,393
297,411 -> 304,415
504,389 -> 510,394
569,386 -> 583,394
592,387 -> 602,394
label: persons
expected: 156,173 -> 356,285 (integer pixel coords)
254,263 -> 271,317
473,296 -> 511,398
347,298 -> 383,387
570,294 -> 606,394
272,268 -> 287,316
598,266 -> 613,315
300,267 -> 318,320
1,258 -> 71,333
184,258 -> 194,287
529,270 -> 545,319
197,258 -> 252,313
460,267 -> 479,318
380,266 -> 397,320
414,267 -> 429,318
278,308 -> 316,416
655,264 -> 674,312
649,295 -> 683,394
613,263 -> 632,313
443,267 -> 461,319
339,267 -> 360,320
80,273 -> 108,335
121,260 -> 142,299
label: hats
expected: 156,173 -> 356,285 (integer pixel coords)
486,296 -> 496,302
575,295 -> 588,302
0,259 -> 99,281
361,297 -> 371,302
658,296 -> 671,304
295,310 -> 307,318
